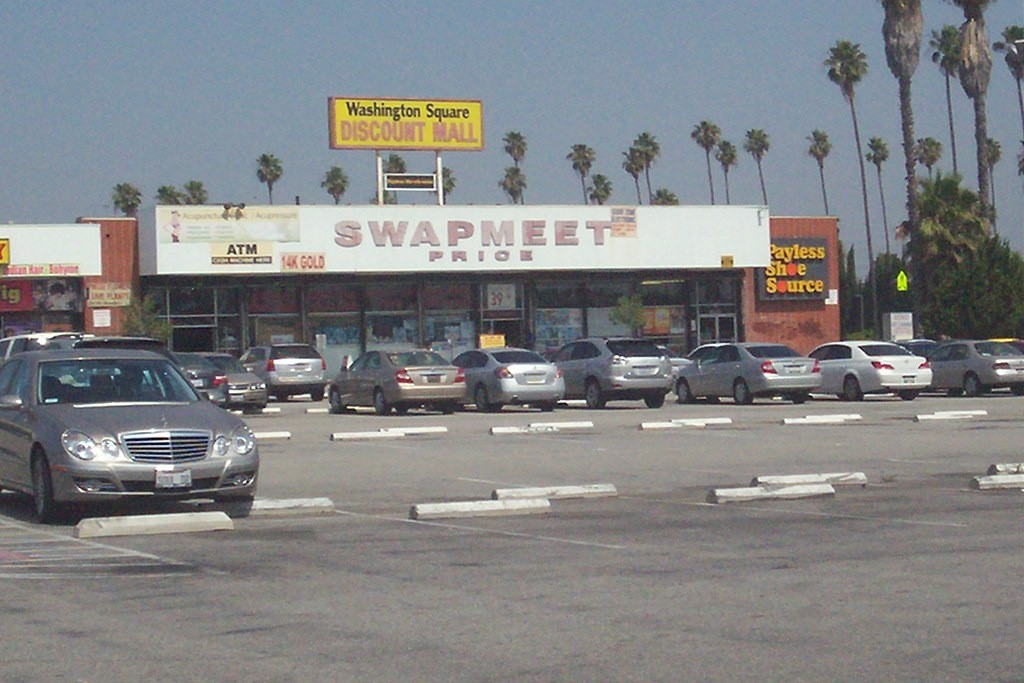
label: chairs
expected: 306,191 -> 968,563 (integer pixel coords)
89,375 -> 115,395
42,377 -> 66,399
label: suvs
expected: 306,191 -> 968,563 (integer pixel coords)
34,337 -> 183,391
239,343 -> 328,401
551,336 -> 673,409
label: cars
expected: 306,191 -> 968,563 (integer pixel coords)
892,339 -> 940,357
200,352 -> 268,413
924,341 -> 1024,396
327,347 -> 466,416
0,332 -> 95,367
0,349 -> 260,519
450,348 -> 565,412
686,344 -> 729,362
654,344 -> 693,375
989,338 -> 1024,354
805,341 -> 934,400
673,343 -> 822,404
172,353 -> 230,411
538,351 -> 548,359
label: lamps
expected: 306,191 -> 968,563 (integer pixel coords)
223,202 -> 232,210
235,202 -> 245,209
